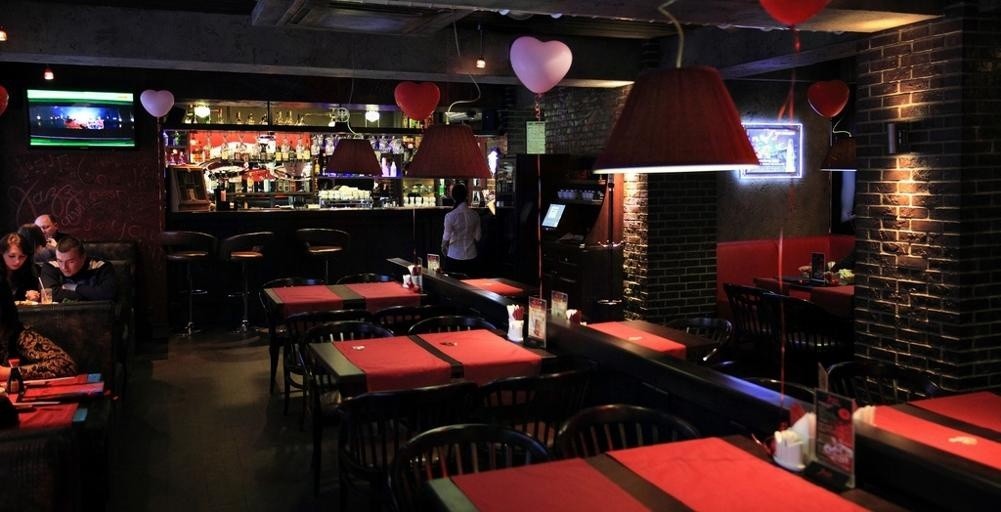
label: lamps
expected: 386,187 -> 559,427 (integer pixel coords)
818,117 -> 858,173
887,121 -> 904,155
589,0 -> 762,176
406,18 -> 492,180
324,54 -> 385,176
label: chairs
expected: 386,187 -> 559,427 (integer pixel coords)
298,227 -> 349,280
157,228 -> 217,339
262,278 -> 734,512
718,271 -> 856,364
222,230 -> 274,338
826,361 -> 947,409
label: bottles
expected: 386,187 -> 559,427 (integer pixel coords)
184,106 -> 309,127
400,176 -> 495,206
160,129 -> 415,209
556,190 -> 597,200
7,357 -> 25,396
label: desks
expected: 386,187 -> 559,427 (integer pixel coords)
429,392 -> 997,511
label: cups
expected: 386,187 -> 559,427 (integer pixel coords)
41,288 -> 53,304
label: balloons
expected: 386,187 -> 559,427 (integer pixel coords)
759,1 -> 830,25
807,79 -> 850,119
510,35 -> 574,95
394,80 -> 441,121
140,89 -> 174,117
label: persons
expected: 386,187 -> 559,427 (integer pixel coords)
0,213 -> 121,383
441,185 -> 482,273
